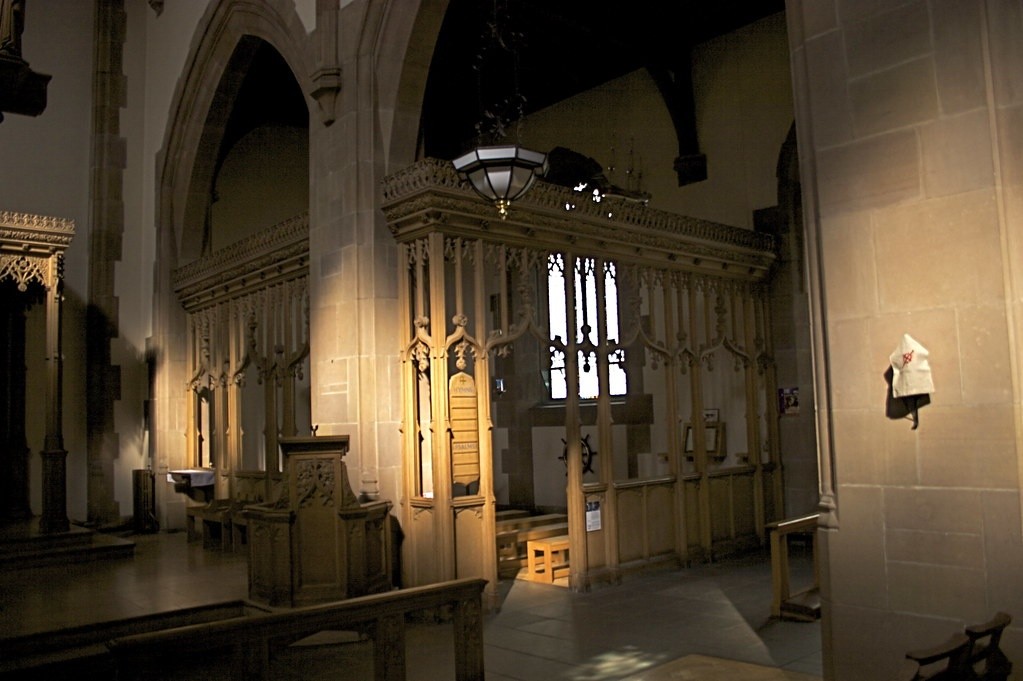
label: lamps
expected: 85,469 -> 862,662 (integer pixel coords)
453,0 -> 551,222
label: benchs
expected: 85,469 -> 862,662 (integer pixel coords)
495,511 -> 570,583
